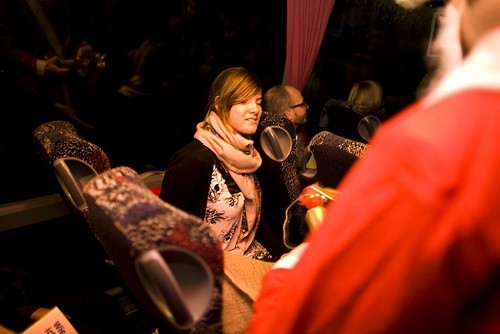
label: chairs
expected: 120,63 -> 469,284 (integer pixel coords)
36,112 -> 366,334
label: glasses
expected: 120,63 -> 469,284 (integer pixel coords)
290,102 -> 305,108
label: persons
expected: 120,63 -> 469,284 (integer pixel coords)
343,83 -> 388,126
262,86 -> 323,222
160,67 -> 273,259
236,0 -> 500,330
